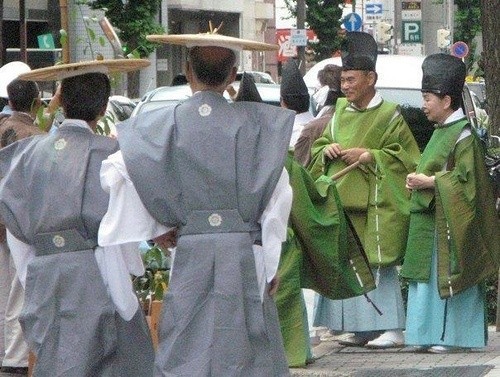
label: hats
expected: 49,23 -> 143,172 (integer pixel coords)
420,53 -> 467,95
340,31 -> 378,72
16,58 -> 151,84
236,72 -> 263,102
147,20 -> 279,53
280,57 -> 308,95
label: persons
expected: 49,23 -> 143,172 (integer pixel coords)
0,61 -> 346,153
402,53 -> 500,352
97,33 -> 296,377
0,78 -> 62,376
305,30 -> 423,349
0,55 -> 155,377
236,68 -> 374,368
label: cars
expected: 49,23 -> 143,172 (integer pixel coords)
304,54 -> 487,153
129,81 -> 314,123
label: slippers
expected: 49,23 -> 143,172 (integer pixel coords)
338,336 -> 371,346
428,347 -> 471,353
364,339 -> 405,348
